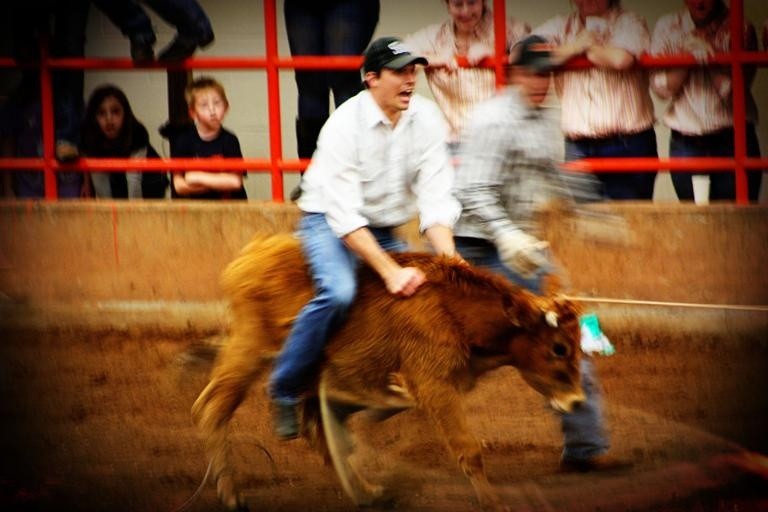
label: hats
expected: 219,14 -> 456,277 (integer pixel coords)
365,36 -> 428,73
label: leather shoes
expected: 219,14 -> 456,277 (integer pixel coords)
262,382 -> 300,442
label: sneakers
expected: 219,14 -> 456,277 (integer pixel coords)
560,454 -> 635,475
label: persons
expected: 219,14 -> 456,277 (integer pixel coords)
284,0 -> 380,200
405,1 -> 768,206
1,1 -> 249,200
262,35 -> 470,441
443,34 -> 636,482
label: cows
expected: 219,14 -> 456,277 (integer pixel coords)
189,227 -> 588,512
